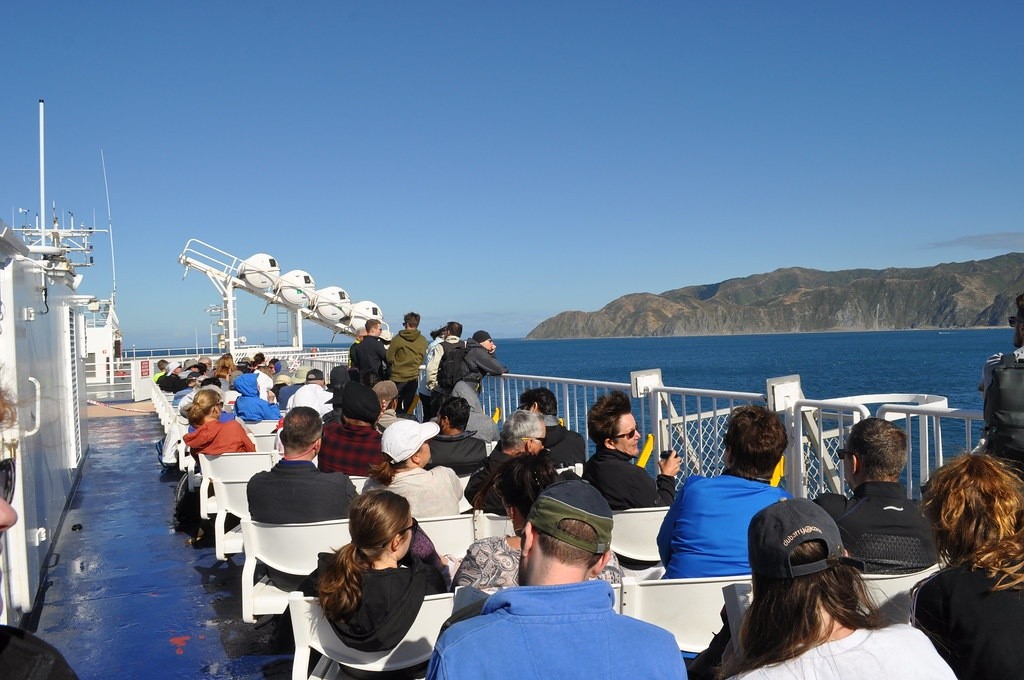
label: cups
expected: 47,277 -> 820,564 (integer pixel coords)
660,450 -> 678,473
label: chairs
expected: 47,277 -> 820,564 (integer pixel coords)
149,383 -> 945,680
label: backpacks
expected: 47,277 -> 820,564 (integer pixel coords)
985,352 -> 1024,456
435,345 -> 482,387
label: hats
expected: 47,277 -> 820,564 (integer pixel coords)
307,368 -> 324,379
343,381 -> 380,422
184,359 -> 198,370
747,497 -> 866,578
275,375 -> 292,385
325,389 -> 344,404
473,330 -> 491,343
165,362 -> 180,376
381,420 -> 440,464
372,380 -> 398,408
526,479 -> 615,554
187,372 -> 200,379
291,365 -> 314,384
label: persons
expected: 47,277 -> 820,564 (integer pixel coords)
0,385 -> 80,680
709,496 -> 958,680
835,417 -> 941,575
157,313 -> 682,570
984,295 -> 1024,480
248,407 -> 359,593
314,489 -> 449,680
449,453 -> 624,593
657,403 -> 794,670
425,480 -> 688,680
909,452 -> 1024,680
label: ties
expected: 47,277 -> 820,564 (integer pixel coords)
267,367 -> 273,369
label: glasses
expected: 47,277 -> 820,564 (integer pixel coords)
382,516 -> 418,548
516,404 -> 529,411
1007,316 -> 1024,328
835,448 -> 856,459
403,322 -> 407,326
214,402 -> 224,409
612,422 -> 640,439
521,436 -> 547,447
487,339 -> 492,343
178,366 -> 181,368
